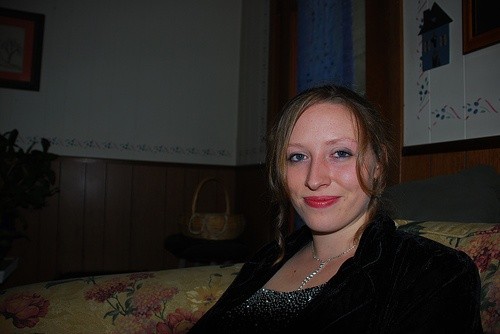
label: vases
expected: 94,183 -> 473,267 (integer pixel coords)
0,129 -> 59,289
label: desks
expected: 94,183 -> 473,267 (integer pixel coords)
168,234 -> 239,267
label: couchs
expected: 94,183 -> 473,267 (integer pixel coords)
0,167 -> 500,334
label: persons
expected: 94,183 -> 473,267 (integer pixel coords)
186,84 -> 484,334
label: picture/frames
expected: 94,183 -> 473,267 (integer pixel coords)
0,6 -> 45,92
462,0 -> 500,54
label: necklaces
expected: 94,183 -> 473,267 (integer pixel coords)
297,240 -> 355,291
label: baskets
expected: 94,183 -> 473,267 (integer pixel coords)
177,175 -> 246,240
